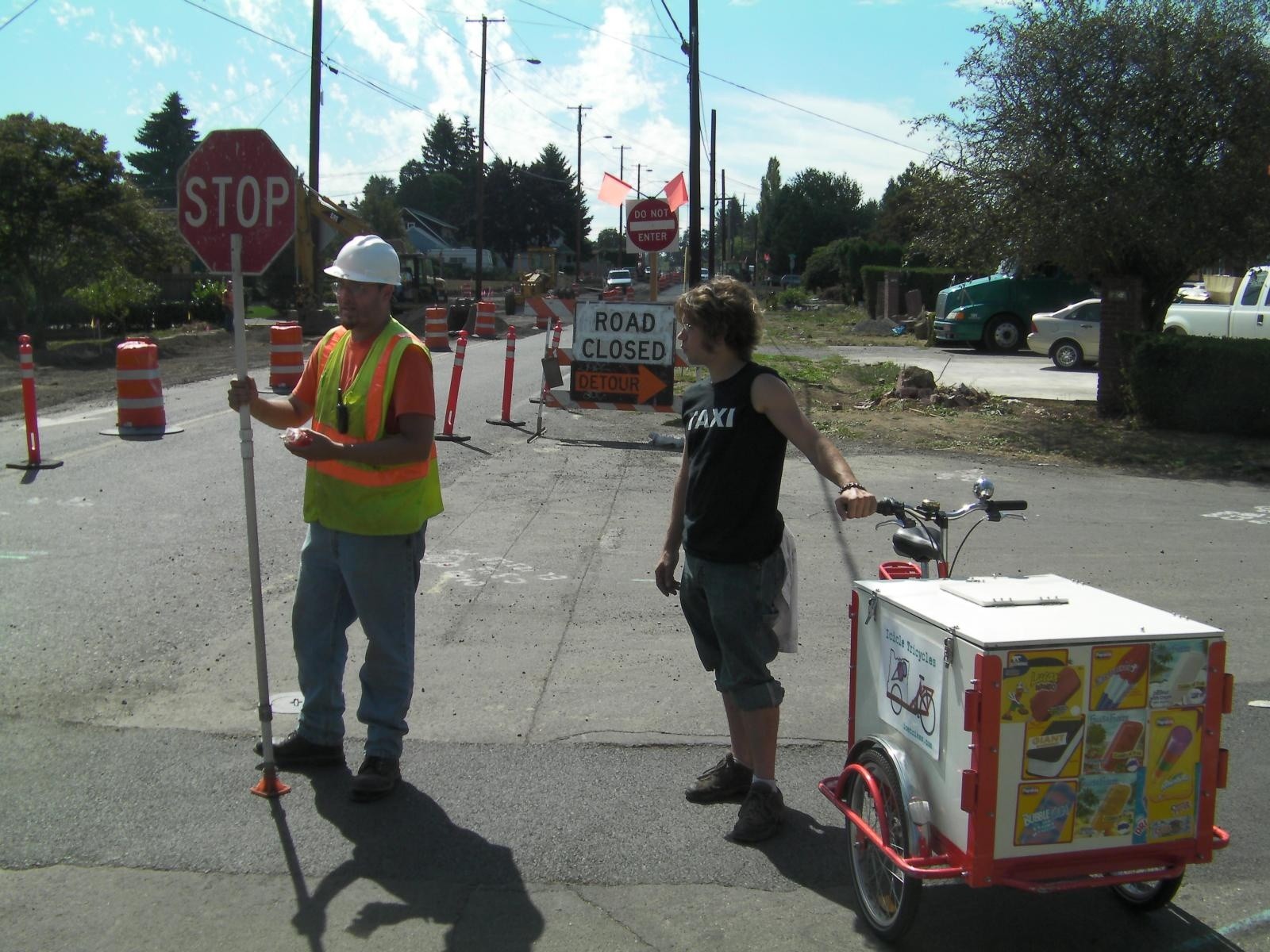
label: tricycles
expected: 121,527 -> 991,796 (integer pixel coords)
834,477 -> 1236,943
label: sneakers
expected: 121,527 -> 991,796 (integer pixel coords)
683,752 -> 762,802
250,729 -> 349,767
731,782 -> 788,843
341,755 -> 400,802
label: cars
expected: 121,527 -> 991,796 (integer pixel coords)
1026,298 -> 1101,369
701,268 -> 709,281
607,268 -> 634,295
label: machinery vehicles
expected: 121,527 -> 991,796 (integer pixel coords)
288,165 -> 452,337
504,244 -> 573,314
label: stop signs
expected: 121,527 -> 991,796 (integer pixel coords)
174,128 -> 300,277
627,199 -> 680,255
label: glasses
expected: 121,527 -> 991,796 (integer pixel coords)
330,280 -> 377,298
682,323 -> 693,333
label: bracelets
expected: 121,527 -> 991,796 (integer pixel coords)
839,483 -> 868,495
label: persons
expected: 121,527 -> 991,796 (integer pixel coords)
228,234 -> 442,799
655,276 -> 878,844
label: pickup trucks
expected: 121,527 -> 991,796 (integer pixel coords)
1162,267 -> 1270,347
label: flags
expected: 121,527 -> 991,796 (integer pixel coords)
598,172 -> 632,208
663,171 -> 689,212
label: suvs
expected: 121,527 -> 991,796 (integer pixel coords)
781,274 -> 803,291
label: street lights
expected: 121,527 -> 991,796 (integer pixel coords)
474,57 -> 545,304
574,135 -> 614,280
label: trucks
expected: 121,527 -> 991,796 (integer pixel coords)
933,266 -> 1102,356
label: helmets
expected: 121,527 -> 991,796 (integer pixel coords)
323,235 -> 402,286
